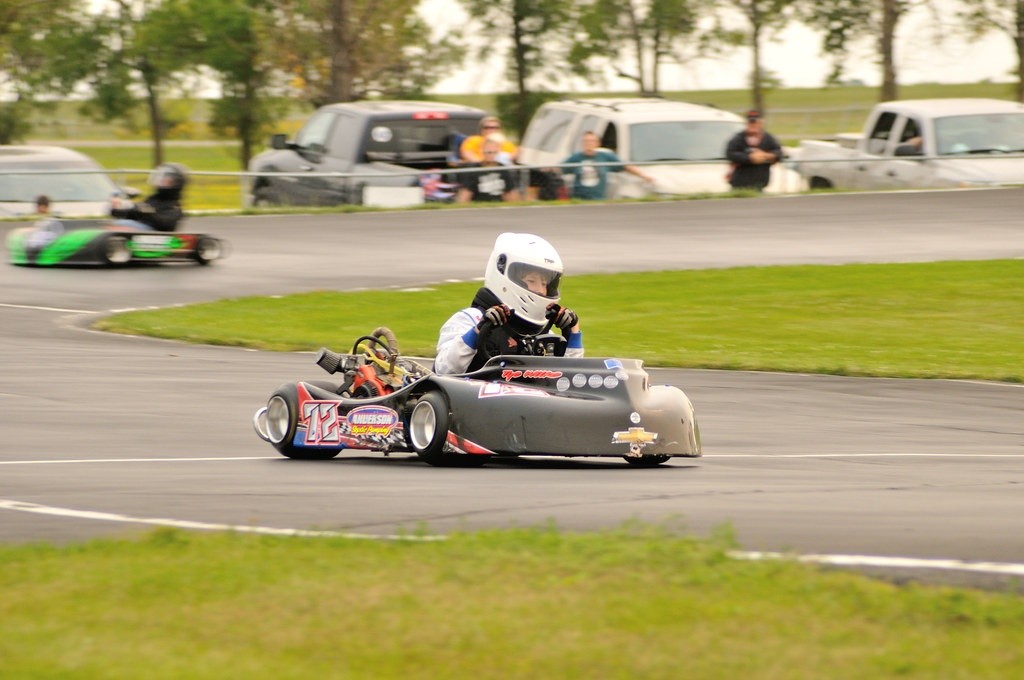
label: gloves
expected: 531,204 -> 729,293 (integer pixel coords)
477,302 -> 510,330
545,301 -> 578,330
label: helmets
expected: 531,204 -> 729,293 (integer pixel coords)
484,231 -> 563,328
148,161 -> 185,191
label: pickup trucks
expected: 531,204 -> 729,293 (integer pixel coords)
800,96 -> 1024,193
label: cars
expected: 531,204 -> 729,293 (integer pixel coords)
0,145 -> 145,220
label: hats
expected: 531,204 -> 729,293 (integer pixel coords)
745,109 -> 764,121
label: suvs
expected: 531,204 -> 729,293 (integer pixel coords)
516,97 -> 809,201
245,101 -> 501,214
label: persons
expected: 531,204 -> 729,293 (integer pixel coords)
457,116 -> 520,167
431,231 -> 585,375
540,131 -> 653,201
110,162 -> 186,232
726,109 -> 783,191
454,138 -> 519,205
32,194 -> 64,235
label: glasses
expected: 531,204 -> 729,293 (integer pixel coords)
481,125 -> 501,130
